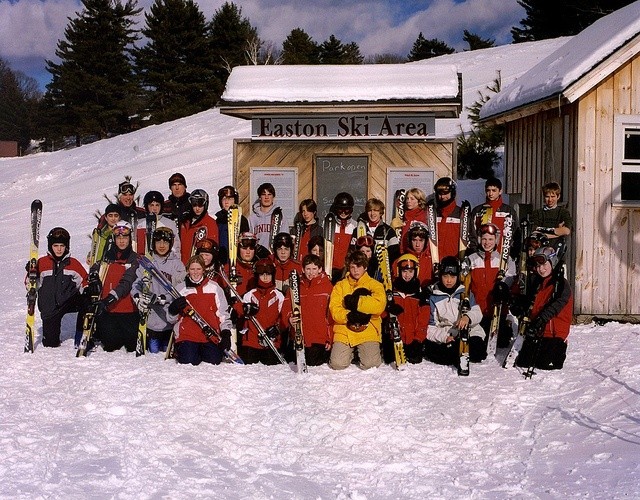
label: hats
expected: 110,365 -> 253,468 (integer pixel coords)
168,173 -> 187,189
219,186 -> 238,207
118,181 -> 136,198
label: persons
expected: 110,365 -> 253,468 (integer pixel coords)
512,231 -> 550,317
164,173 -> 190,214
321,192 -> 358,268
399,188 -> 426,254
392,220 -> 439,290
340,236 -> 384,284
289,199 -> 323,264
132,191 -> 182,259
516,246 -> 572,370
196,238 -> 222,287
233,258 -> 285,366
462,224 -> 519,348
328,251 -> 387,369
89,219 -> 139,352
256,232 -> 302,292
282,254 -> 334,366
180,189 -> 218,266
219,232 -> 260,306
97,176 -> 145,229
426,177 -> 461,264
215,186 -> 249,264
307,235 -> 342,286
358,198 -> 399,268
165,255 -> 232,365
426,256 -> 488,376
248,183 -> 289,255
24,227 -> 95,348
382,254 -> 430,364
131,227 -> 186,354
467,177 -> 522,264
527,182 -> 573,280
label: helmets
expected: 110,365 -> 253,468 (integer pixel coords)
437,256 -> 461,277
273,232 -> 294,250
534,247 -> 558,271
196,239 -> 219,257
152,226 -> 175,247
333,193 -> 354,208
112,220 -> 132,230
407,222 -> 430,243
47,227 -> 70,240
477,223 -> 501,245
252,258 -> 275,276
239,232 -> 257,249
143,191 -> 164,203
189,189 -> 209,206
434,177 -> 457,193
396,253 -> 420,277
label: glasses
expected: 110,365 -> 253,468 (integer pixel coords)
274,239 -> 293,247
438,266 -> 461,274
526,252 -> 556,266
218,189 -> 238,197
408,229 -> 429,237
478,225 -> 500,234
356,237 -> 376,247
195,241 -> 218,250
335,208 -> 352,214
153,231 -> 175,241
239,238 -> 257,247
119,183 -> 135,194
113,227 -> 132,236
48,229 -> 70,240
188,196 -> 206,205
254,265 -> 275,274
396,259 -> 419,269
434,188 -> 452,195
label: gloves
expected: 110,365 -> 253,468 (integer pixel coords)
244,302 -> 258,316
169,297 -> 187,315
266,322 -> 280,338
219,330 -> 231,352
344,294 -> 357,309
87,273 -> 102,295
390,304 -> 404,315
91,300 -> 108,317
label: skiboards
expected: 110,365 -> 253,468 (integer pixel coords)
391,188 -> 407,241
426,200 -> 440,281
289,270 -> 308,373
292,222 -> 304,260
187,225 -> 209,264
518,217 -> 531,294
323,212 -> 335,279
270,207 -> 284,253
481,205 -> 493,224
487,215 -> 513,355
90,228 -> 101,267
375,243 -> 406,371
76,233 -> 114,357
355,218 -> 367,239
503,240 -> 567,367
458,200 -> 471,261
226,203 -> 242,323
136,211 -> 156,356
24,199 -> 42,353
140,256 -> 244,363
212,263 -> 287,363
458,256 -> 471,375
